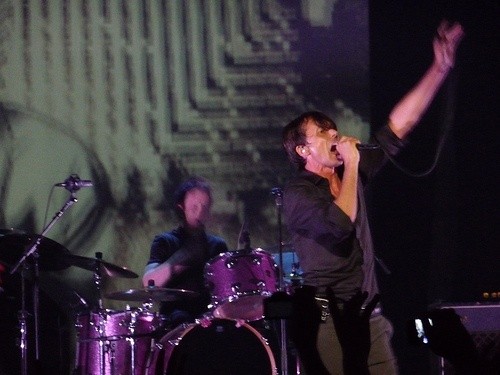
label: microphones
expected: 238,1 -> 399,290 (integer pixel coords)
238,223 -> 246,249
270,187 -> 284,206
357,144 -> 374,151
57,180 -> 93,188
73,290 -> 90,309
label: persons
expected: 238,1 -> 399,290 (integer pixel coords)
283,19 -> 465,375
143,173 -> 251,331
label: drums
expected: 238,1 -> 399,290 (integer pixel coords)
205,247 -> 278,322
72,309 -> 164,375
151,317 -> 279,375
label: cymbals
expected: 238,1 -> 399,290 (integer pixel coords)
68,253 -> 139,278
0,229 -> 73,270
103,287 -> 196,303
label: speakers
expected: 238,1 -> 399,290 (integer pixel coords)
440,304 -> 500,375
